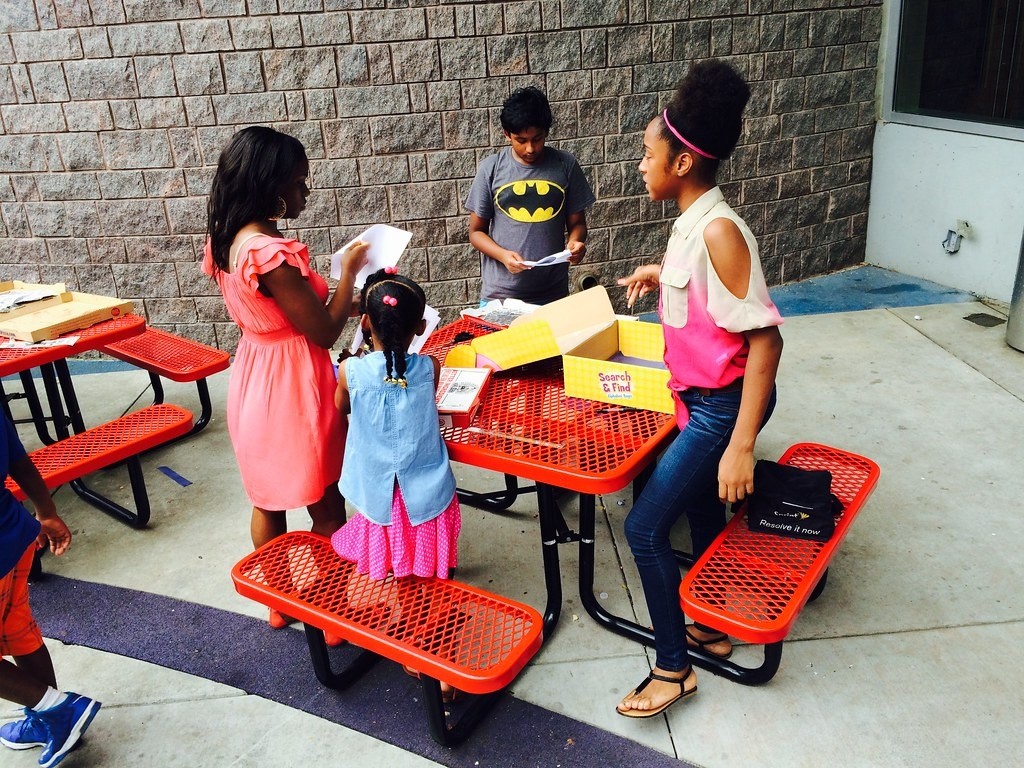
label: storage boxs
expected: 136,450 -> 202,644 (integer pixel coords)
0,280 -> 135,344
445,285 -> 677,415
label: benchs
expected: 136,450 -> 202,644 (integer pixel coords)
231,530 -> 543,747
120,328 -> 230,430
614,444 -> 881,689
4,404 -> 194,528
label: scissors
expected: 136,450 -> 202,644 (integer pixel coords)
438,331 -> 475,349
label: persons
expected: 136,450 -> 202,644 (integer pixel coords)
0,378 -> 102,768
617,60 -> 784,718
332,267 -> 461,703
465,87 -> 597,309
202,125 -> 390,645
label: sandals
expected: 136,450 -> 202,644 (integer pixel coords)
616,664 -> 697,718
648,622 -> 733,660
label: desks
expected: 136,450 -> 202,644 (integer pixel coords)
415,315 -> 677,633
0,312 -> 147,499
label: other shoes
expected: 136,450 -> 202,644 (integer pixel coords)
324,601 -> 391,647
269,580 -> 323,629
402,662 -> 457,704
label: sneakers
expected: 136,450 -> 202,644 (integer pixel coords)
24,691 -> 102,768
0,706 -> 51,751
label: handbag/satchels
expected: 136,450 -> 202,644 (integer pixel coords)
731,459 -> 844,542
